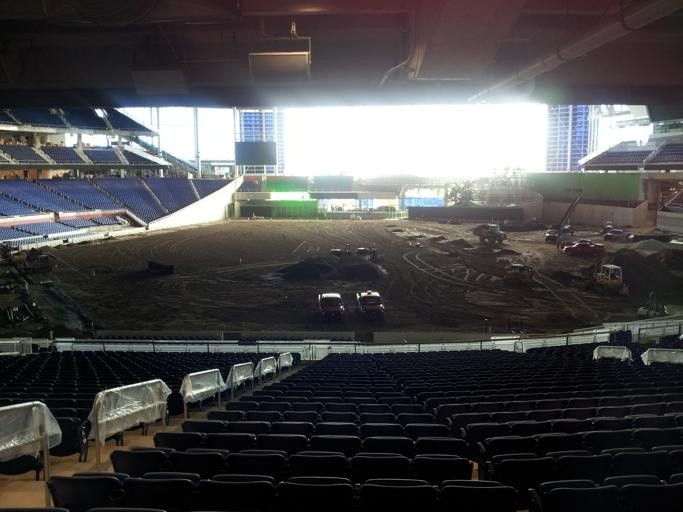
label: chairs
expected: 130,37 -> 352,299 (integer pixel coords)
0,341 -> 683,511
660,334 -> 682,348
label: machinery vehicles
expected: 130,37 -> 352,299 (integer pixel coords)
545,194 -> 581,242
584,264 -> 622,295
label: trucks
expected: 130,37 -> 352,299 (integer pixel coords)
472,224 -> 505,244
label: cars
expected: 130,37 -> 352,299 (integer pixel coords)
317,292 -> 344,317
562,240 -> 604,257
355,290 -> 384,315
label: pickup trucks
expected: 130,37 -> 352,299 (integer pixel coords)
604,227 -> 631,242
